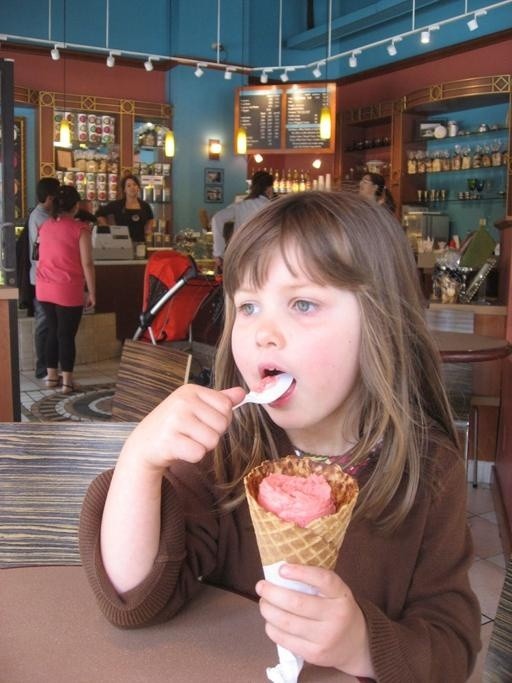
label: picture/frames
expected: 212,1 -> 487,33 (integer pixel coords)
204,168 -> 225,204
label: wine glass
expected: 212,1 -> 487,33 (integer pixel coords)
467,178 -> 493,199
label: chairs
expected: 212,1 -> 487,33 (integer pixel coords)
109,338 -> 192,425
440,362 -> 479,489
0,422 -> 143,567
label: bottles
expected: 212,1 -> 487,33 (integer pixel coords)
479,123 -> 488,132
350,167 -> 354,180
406,139 -> 507,174
250,167 -> 331,194
448,121 -> 458,137
417,188 -> 447,201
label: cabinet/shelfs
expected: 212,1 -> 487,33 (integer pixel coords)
395,74 -> 511,314
335,99 -> 396,196
243,150 -> 336,197
41,91 -> 174,253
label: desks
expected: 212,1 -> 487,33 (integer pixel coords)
1,568 -> 362,683
426,328 -> 511,362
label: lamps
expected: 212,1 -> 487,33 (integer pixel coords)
318,2 -> 332,141
59,0 -> 69,148
164,0 -> 176,158
0,0 -> 511,84
237,0 -> 247,156
209,139 -> 220,160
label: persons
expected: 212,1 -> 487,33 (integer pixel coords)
106,175 -> 155,243
27,177 -> 60,386
357,171 -> 396,215
211,171 -> 275,271
35,184 -> 96,396
78,180 -> 484,683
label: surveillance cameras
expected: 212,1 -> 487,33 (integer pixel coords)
212,42 -> 224,51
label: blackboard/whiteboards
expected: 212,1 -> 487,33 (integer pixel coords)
235,83 -> 336,154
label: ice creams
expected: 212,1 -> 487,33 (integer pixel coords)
242,454 -> 360,683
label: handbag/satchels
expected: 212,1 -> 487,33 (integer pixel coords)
32,241 -> 39,261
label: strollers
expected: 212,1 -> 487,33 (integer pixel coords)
129,245 -> 227,384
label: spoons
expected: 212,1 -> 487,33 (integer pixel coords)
231,371 -> 293,410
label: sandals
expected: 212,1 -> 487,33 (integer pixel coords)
46,376 -> 82,394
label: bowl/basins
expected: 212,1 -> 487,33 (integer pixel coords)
458,226 -> 495,269
